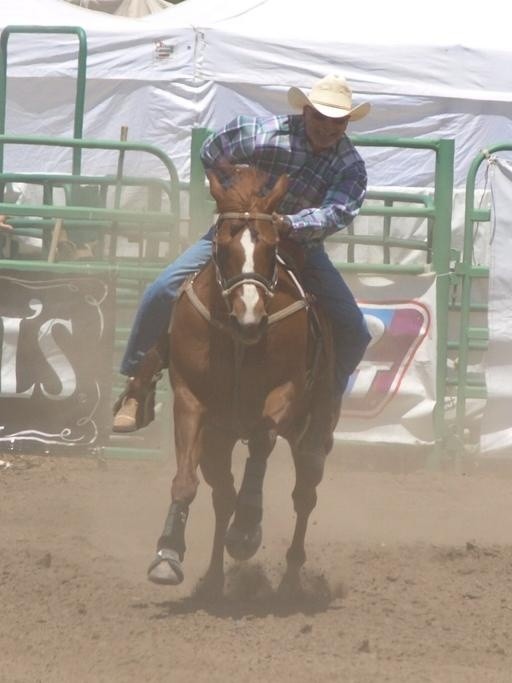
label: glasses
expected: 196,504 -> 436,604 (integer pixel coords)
305,103 -> 347,125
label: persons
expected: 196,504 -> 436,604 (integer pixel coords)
110,71 -> 373,434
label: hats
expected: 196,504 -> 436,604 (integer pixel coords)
285,72 -> 370,121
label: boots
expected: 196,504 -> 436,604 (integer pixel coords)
109,372 -> 162,435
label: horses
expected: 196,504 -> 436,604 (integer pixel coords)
146,157 -> 342,604
